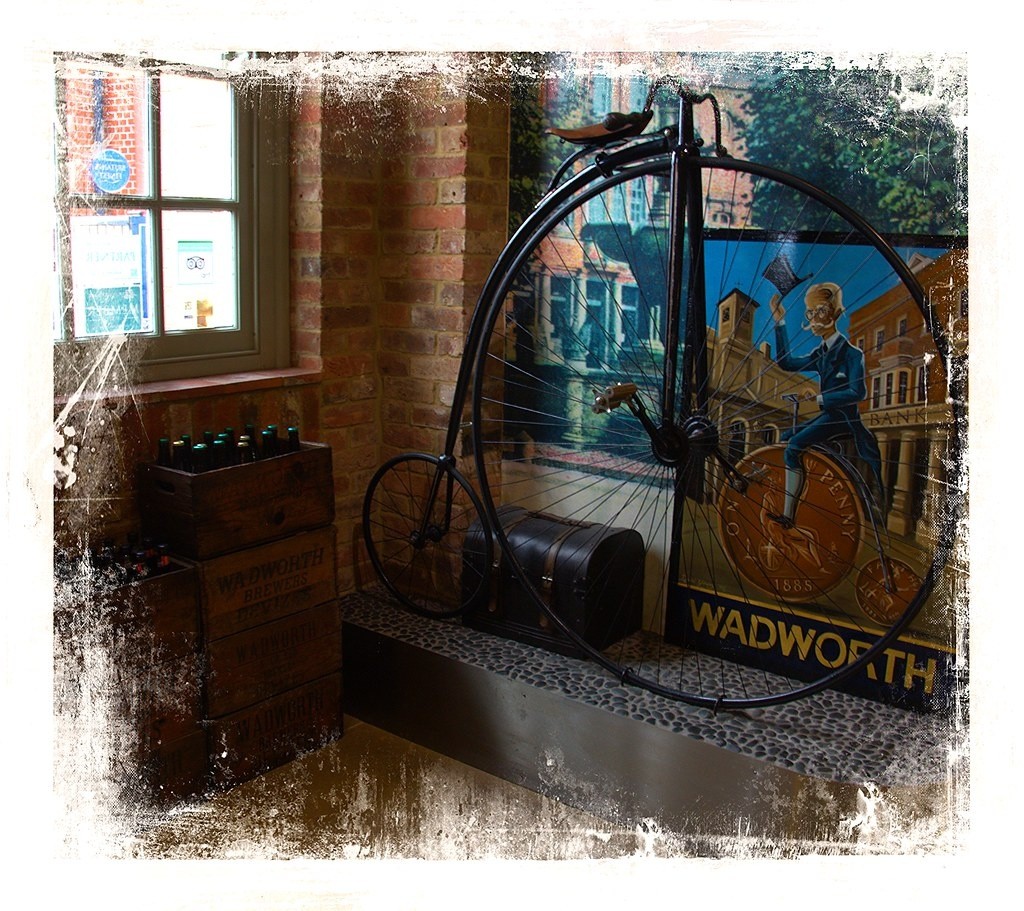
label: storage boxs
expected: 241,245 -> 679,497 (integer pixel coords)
53,440 -> 346,812
460,504 -> 645,660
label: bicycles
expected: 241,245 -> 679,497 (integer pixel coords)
358,72 -> 964,715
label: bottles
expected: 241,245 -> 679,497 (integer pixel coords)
157,425 -> 301,474
88,538 -> 172,583
